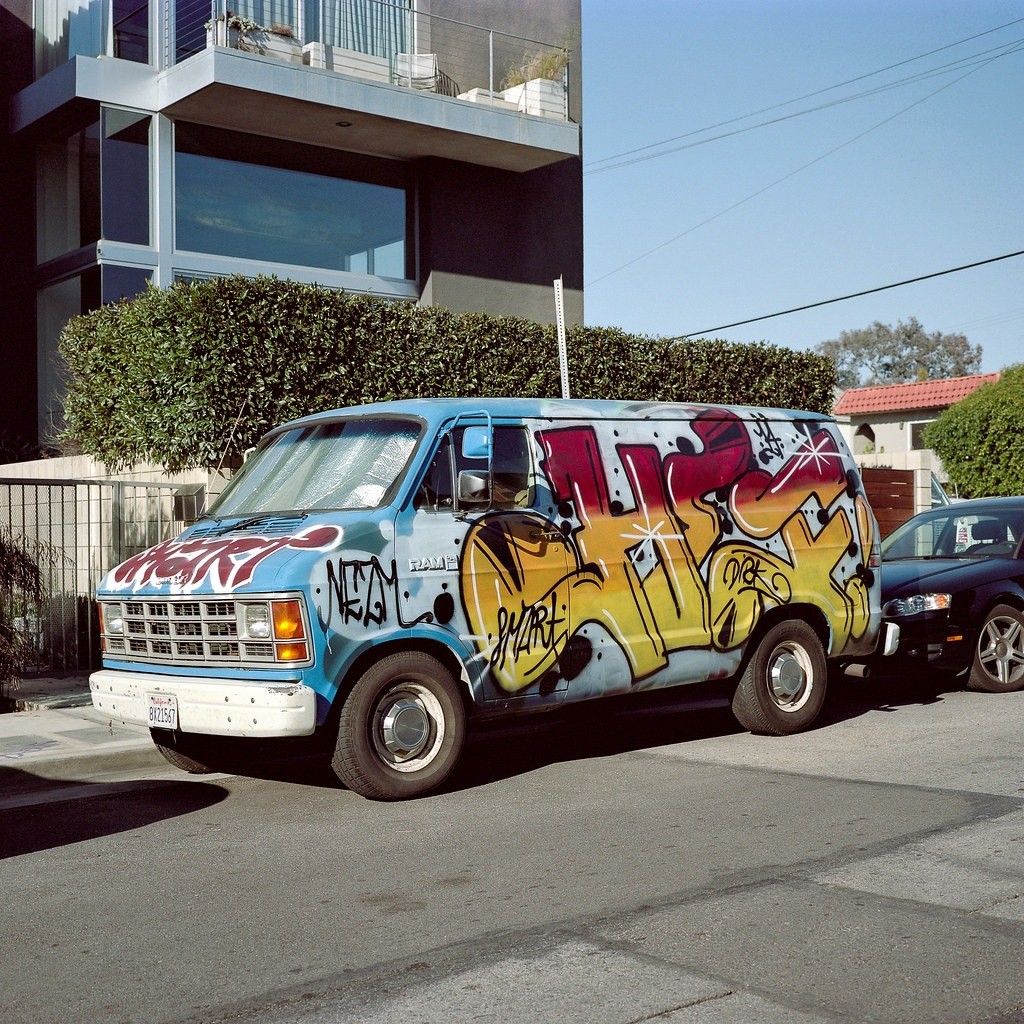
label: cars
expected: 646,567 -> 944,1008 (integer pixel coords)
873,494 -> 1024,693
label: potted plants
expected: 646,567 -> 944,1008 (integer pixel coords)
205,11 -> 305,65
497,35 -> 576,121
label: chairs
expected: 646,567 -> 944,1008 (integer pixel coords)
963,519 -> 1004,554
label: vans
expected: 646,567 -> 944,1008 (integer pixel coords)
87,395 -> 883,803
